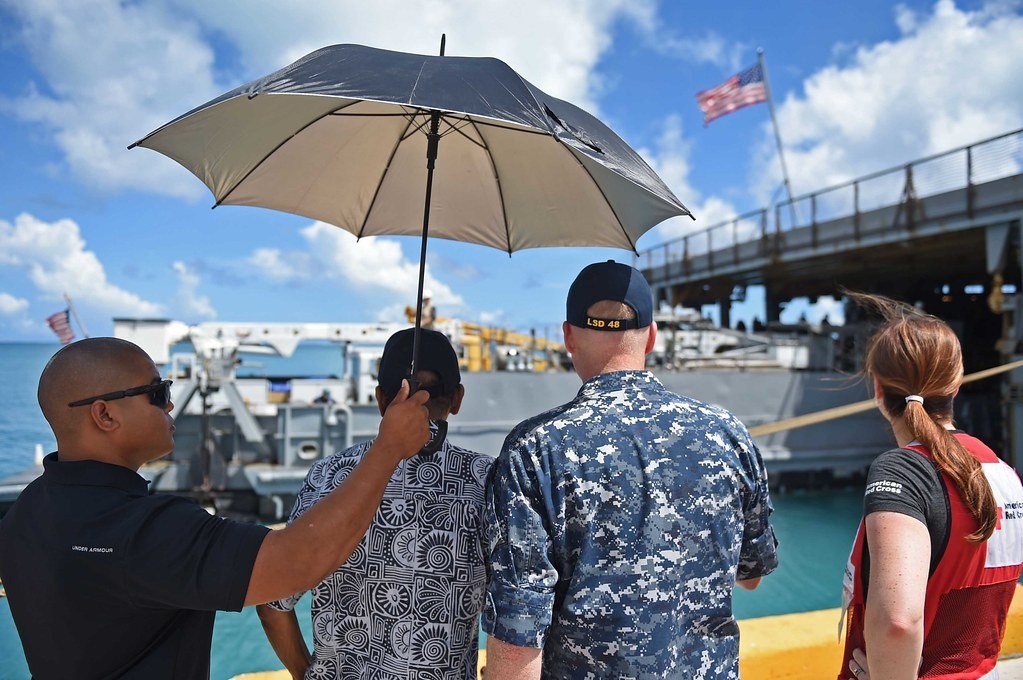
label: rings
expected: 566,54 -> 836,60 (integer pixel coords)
854,668 -> 860,676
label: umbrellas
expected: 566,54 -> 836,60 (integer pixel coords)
128,42 -> 696,456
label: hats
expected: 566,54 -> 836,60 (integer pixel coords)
378,328 -> 461,396
567,261 -> 653,331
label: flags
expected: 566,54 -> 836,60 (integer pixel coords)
695,63 -> 766,125
46,310 -> 75,344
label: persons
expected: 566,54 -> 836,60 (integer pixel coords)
836,291 -> 1023,680
255,328 -> 497,680
314,389 -> 334,404
479,259 -> 778,680
1,335 -> 433,679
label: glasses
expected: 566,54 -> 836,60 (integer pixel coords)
68,378 -> 174,407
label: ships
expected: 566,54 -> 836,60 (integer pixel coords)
0,299 -> 973,528
